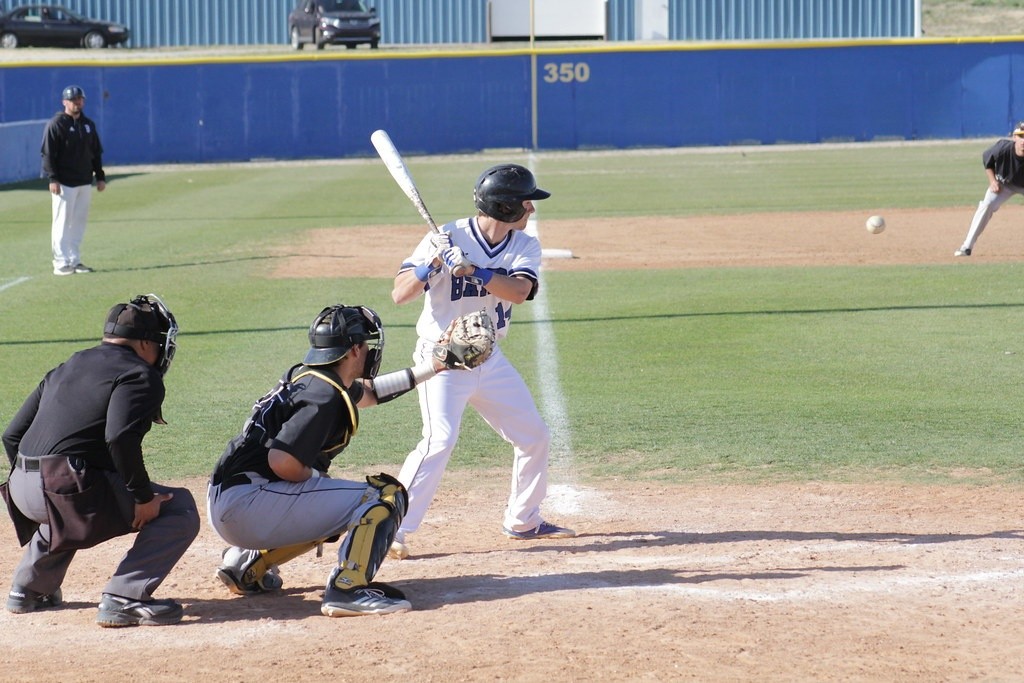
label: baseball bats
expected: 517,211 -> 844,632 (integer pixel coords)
370,129 -> 467,279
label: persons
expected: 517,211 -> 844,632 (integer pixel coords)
382,164 -> 575,558
209,304 -> 494,616
1,293 -> 201,628
954,121 -> 1024,255
40,85 -> 106,275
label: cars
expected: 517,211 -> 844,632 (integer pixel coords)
286,0 -> 383,49
0,6 -> 129,49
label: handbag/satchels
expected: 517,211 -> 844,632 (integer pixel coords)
40,455 -> 130,554
0,456 -> 39,548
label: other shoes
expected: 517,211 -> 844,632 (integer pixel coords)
955,247 -> 972,257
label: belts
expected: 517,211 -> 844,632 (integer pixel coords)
14,456 -> 39,471
216,474 -> 251,498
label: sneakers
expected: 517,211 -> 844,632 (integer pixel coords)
96,593 -> 184,627
503,520 -> 575,540
218,567 -> 283,595
6,582 -> 62,614
385,539 -> 410,559
322,585 -> 412,617
75,264 -> 93,274
53,266 -> 75,276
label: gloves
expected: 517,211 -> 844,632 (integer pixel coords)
442,246 -> 475,276
428,233 -> 450,259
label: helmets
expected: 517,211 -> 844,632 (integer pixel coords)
62,84 -> 87,100
474,165 -> 551,223
301,305 -> 369,366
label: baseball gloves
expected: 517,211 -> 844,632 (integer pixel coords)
431,307 -> 495,372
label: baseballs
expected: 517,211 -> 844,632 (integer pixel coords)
866,216 -> 886,235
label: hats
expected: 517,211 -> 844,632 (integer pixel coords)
102,302 -> 176,344
1013,122 -> 1024,135
